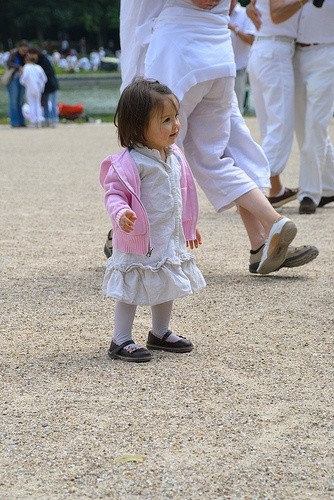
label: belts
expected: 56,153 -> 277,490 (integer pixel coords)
295,42 -> 321,47
253,35 -> 296,44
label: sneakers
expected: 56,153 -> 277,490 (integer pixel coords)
103,229 -> 113,260
249,241 -> 319,273
266,187 -> 299,208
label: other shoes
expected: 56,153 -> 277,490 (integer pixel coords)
256,215 -> 297,274
108,338 -> 152,363
146,330 -> 194,353
317,196 -> 334,208
299,196 -> 316,215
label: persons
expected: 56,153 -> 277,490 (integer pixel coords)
5,39 -> 30,128
222,0 -> 334,214
27,47 -> 61,129
43,40 -> 105,72
102,1 -> 318,275
19,52 -> 48,128
96,77 -> 207,361
